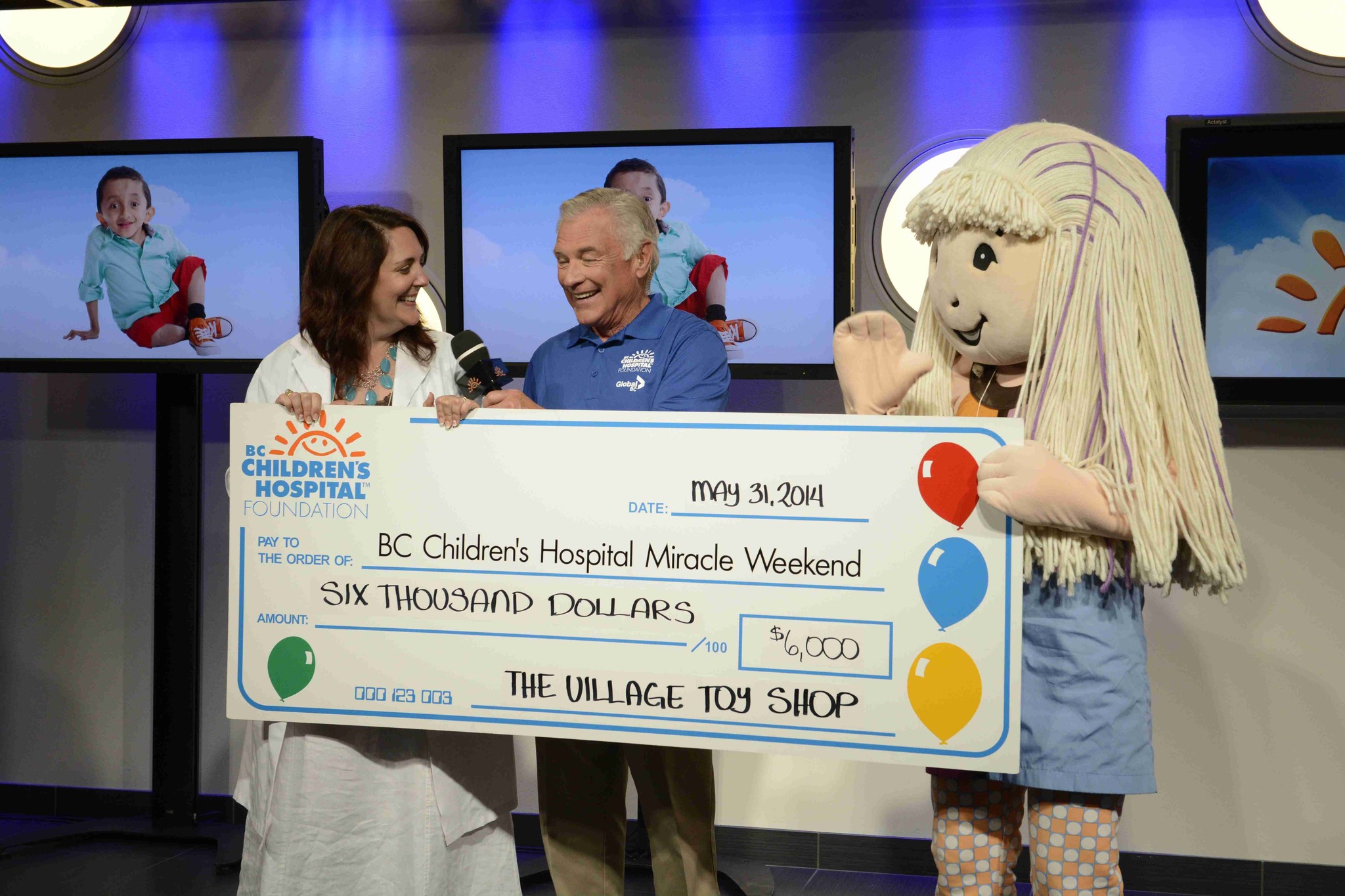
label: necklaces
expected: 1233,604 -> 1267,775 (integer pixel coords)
334,343 -> 398,405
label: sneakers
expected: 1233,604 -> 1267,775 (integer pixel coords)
188,317 -> 231,341
186,317 -> 221,357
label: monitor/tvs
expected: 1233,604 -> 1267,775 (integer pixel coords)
1164,108 -> 1345,419
0,135 -> 324,374
441,125 -> 857,379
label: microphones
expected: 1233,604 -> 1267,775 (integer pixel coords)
450,330 -> 515,400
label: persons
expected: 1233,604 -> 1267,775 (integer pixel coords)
483,188 -> 732,896
832,120 -> 1248,896
61,165 -> 234,355
232,204 -> 521,896
603,156 -> 758,359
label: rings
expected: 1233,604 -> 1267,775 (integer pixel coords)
506,401 -> 516,410
458,396 -> 466,401
285,389 -> 293,395
